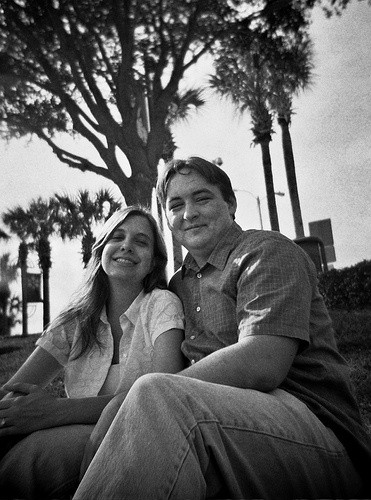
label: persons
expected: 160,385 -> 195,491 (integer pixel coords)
0,156 -> 371,500
0,205 -> 187,460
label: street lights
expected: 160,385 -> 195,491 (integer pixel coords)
232,189 -> 286,231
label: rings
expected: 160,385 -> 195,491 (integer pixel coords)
1,418 -> 6,427
